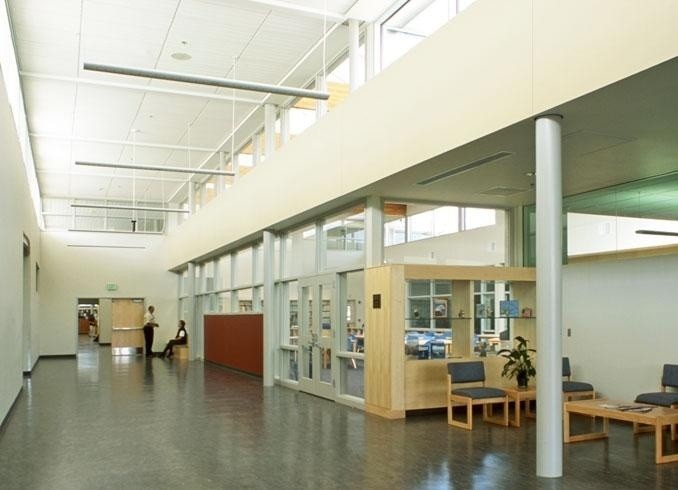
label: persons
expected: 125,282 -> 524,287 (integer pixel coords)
158,320 -> 186,358
347,327 -> 359,354
143,306 -> 159,360
88,313 -> 96,338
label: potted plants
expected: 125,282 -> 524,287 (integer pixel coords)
496,335 -> 536,389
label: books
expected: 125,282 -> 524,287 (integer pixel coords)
598,401 -> 659,413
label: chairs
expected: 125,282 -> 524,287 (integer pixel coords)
447,361 -> 508,430
633,364 -> 678,441
563,357 -> 596,417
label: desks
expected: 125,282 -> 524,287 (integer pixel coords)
488,385 -> 537,428
563,400 -> 678,465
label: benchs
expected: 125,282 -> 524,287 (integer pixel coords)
172,344 -> 190,359
